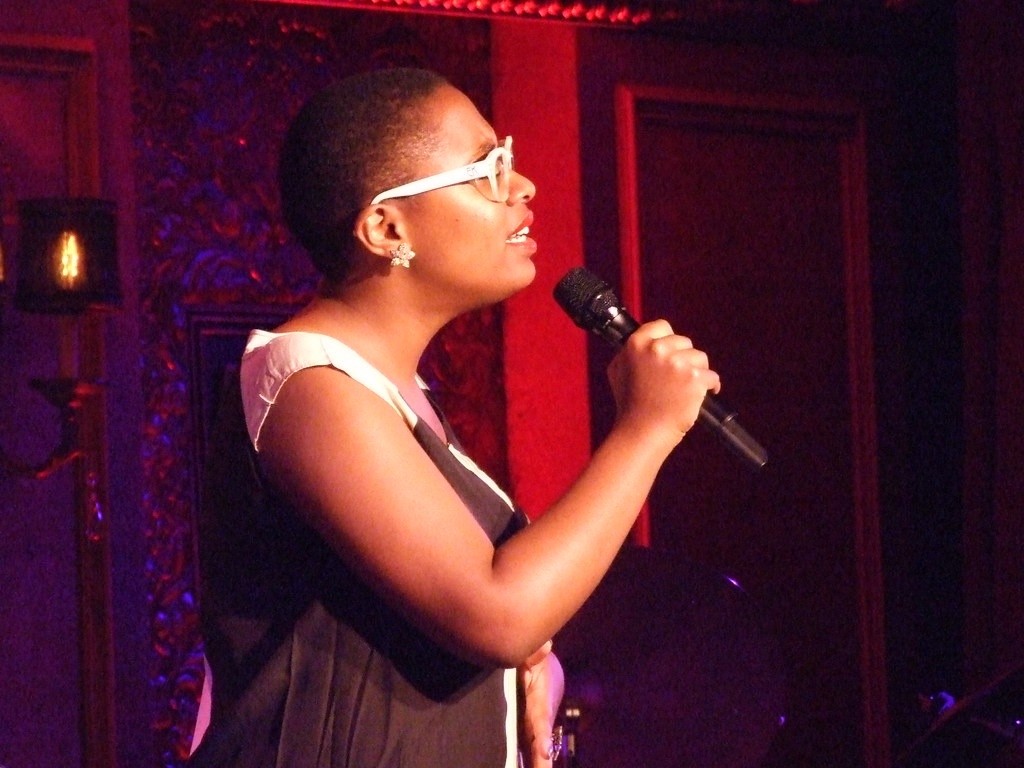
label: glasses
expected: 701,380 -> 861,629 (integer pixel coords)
353,136 -> 514,237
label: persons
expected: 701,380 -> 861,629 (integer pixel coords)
901,689 -> 1000,768
188,66 -> 722,768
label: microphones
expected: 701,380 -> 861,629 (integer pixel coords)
552,264 -> 775,476
